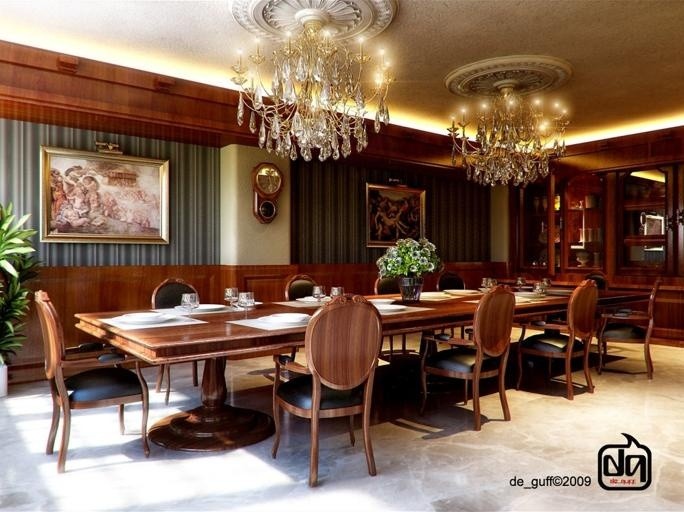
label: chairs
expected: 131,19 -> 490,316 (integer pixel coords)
32,268 -> 664,489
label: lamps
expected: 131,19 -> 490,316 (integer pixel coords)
228,0 -> 401,163
442,55 -> 576,189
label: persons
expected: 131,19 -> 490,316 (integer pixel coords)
367,193 -> 419,240
48,164 -> 105,230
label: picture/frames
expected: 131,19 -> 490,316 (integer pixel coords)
38,140 -> 170,246
364,182 -> 429,248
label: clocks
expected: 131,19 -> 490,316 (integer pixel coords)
250,161 -> 285,224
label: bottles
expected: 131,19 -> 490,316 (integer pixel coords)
577,224 -> 602,242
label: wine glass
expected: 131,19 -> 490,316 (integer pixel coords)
516,276 -> 551,298
312,286 -> 326,309
481,278 -> 497,292
533,196 -> 548,214
180,293 -> 199,323
223,287 -> 238,313
576,251 -> 590,268
237,292 -> 255,320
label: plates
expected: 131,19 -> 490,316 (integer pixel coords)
443,289 -> 478,295
233,301 -> 263,307
546,289 -> 572,296
367,298 -> 407,312
174,304 -> 225,312
296,295 -> 330,302
256,316 -> 312,325
118,312 -> 176,324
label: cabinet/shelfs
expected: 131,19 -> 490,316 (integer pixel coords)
507,125 -> 684,349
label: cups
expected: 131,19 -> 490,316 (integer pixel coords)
584,194 -> 597,209
593,252 -> 600,267
330,287 -> 344,299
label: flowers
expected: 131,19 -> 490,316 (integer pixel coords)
375,236 -> 445,281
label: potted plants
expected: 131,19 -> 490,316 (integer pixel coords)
0,200 -> 44,398
